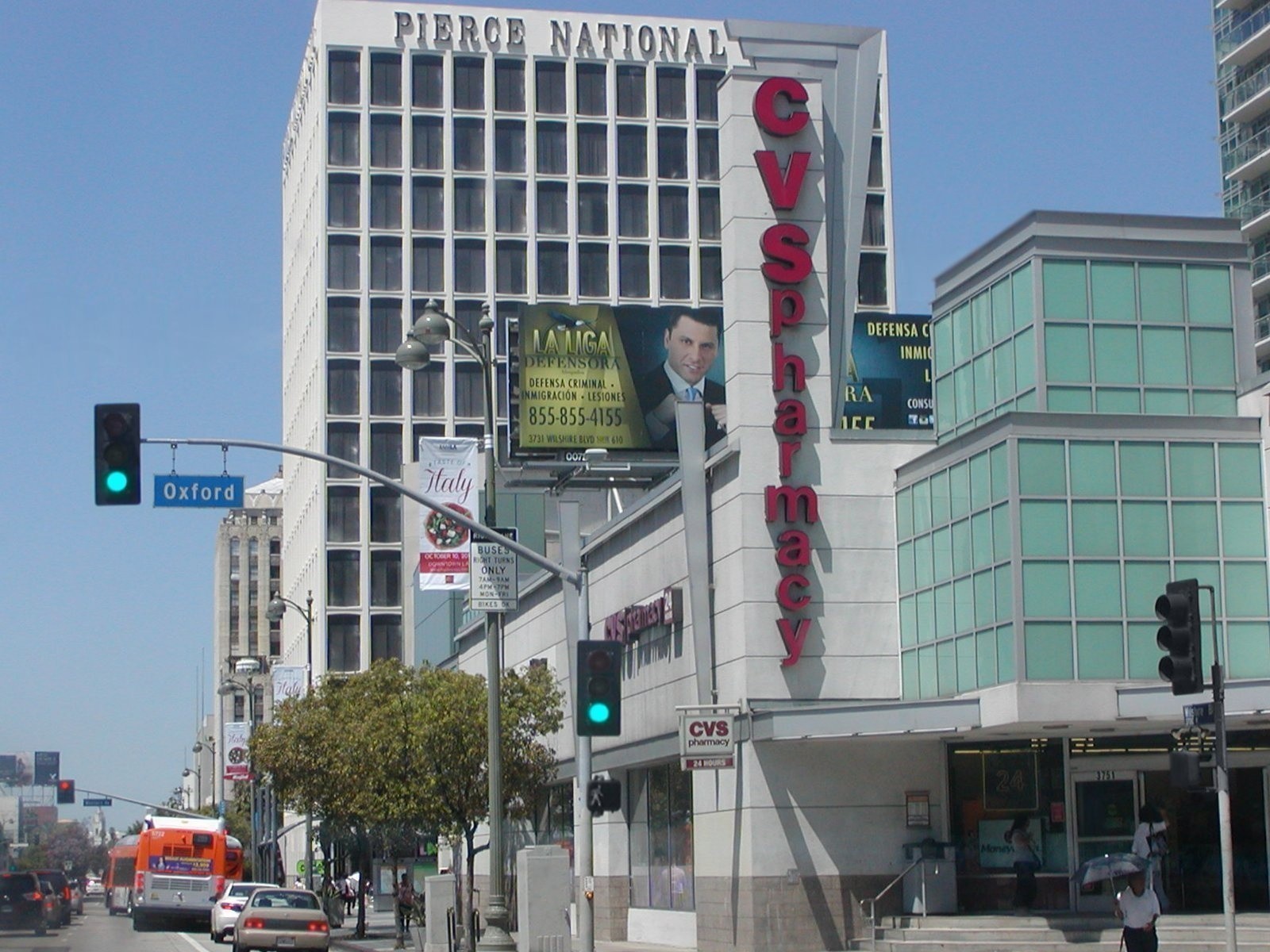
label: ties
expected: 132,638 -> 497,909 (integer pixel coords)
686,385 -> 698,402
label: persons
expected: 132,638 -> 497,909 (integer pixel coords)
660,857 -> 687,909
1115,869 -> 1160,952
291,868 -> 370,916
1011,813 -> 1038,916
1131,803 -> 1175,915
636,306 -> 727,451
398,873 -> 423,932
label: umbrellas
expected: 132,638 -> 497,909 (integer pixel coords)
1070,852 -> 1154,899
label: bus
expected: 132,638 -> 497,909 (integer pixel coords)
104,834 -> 140,915
131,815 -> 227,932
225,834 -> 243,882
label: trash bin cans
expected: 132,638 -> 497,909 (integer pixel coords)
328,888 -> 344,928
901,837 -> 959,915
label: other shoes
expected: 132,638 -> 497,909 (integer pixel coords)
1013,908 -> 1033,917
406,929 -> 410,933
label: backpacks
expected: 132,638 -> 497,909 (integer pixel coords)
399,883 -> 413,909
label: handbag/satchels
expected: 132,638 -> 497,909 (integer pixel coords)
1145,828 -> 1172,857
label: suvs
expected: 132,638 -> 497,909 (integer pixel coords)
24,869 -> 79,927
0,869 -> 53,938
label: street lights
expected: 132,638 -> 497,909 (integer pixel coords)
265,590 -> 314,889
394,295 -> 517,951
216,676 -> 256,883
181,767 -> 202,810
173,787 -> 191,810
192,741 -> 216,820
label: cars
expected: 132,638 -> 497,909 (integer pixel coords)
39,880 -> 65,929
229,887 -> 331,952
86,878 -> 106,897
209,881 -> 288,943
69,880 -> 84,916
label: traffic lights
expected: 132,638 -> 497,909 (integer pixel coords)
57,779 -> 74,804
1153,578 -> 1203,696
587,776 -> 612,813
1170,749 -> 1200,787
94,403 -> 142,507
576,639 -> 621,734
